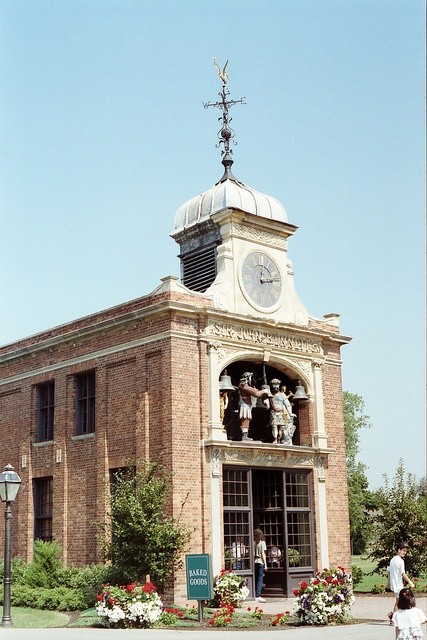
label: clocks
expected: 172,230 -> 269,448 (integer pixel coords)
237,249 -> 289,315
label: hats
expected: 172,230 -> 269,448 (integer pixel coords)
395,543 -> 410,548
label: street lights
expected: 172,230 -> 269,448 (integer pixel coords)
0,463 -> 21,626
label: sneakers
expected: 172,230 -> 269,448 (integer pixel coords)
390,621 -> 394,624
388,611 -> 392,618
254,596 -> 266,603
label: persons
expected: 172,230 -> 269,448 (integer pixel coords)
270,378 -> 297,445
387,543 -> 415,625
281,385 -> 297,445
392,588 -> 427,640
270,546 -> 281,568
252,529 -> 268,602
232,535 -> 249,569
238,372 -> 274,440
